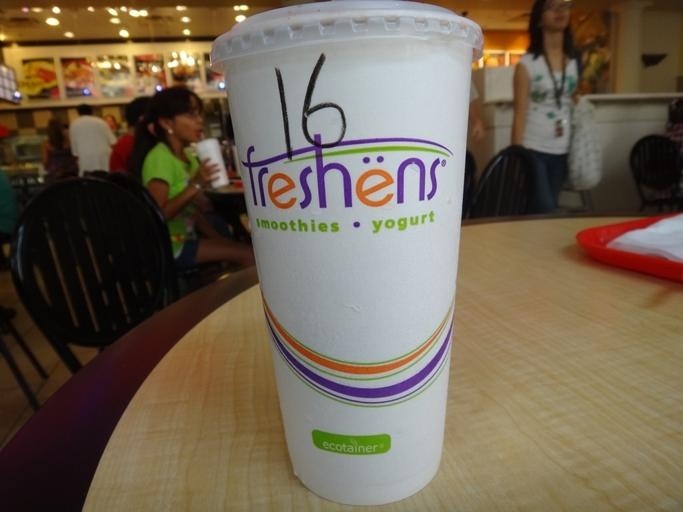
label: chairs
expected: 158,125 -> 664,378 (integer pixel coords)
462,146 -> 532,218
629,134 -> 683,212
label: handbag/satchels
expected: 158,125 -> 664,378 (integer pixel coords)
565,93 -> 617,191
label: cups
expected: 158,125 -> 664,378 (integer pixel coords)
193,137 -> 229,191
213,0 -> 482,507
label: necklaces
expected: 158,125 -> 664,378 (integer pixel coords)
540,47 -> 568,137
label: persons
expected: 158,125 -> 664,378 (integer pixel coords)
651,96 -> 683,198
511,0 -> 583,212
462,81 -> 485,220
581,35 -> 619,94
0,86 -> 257,269
